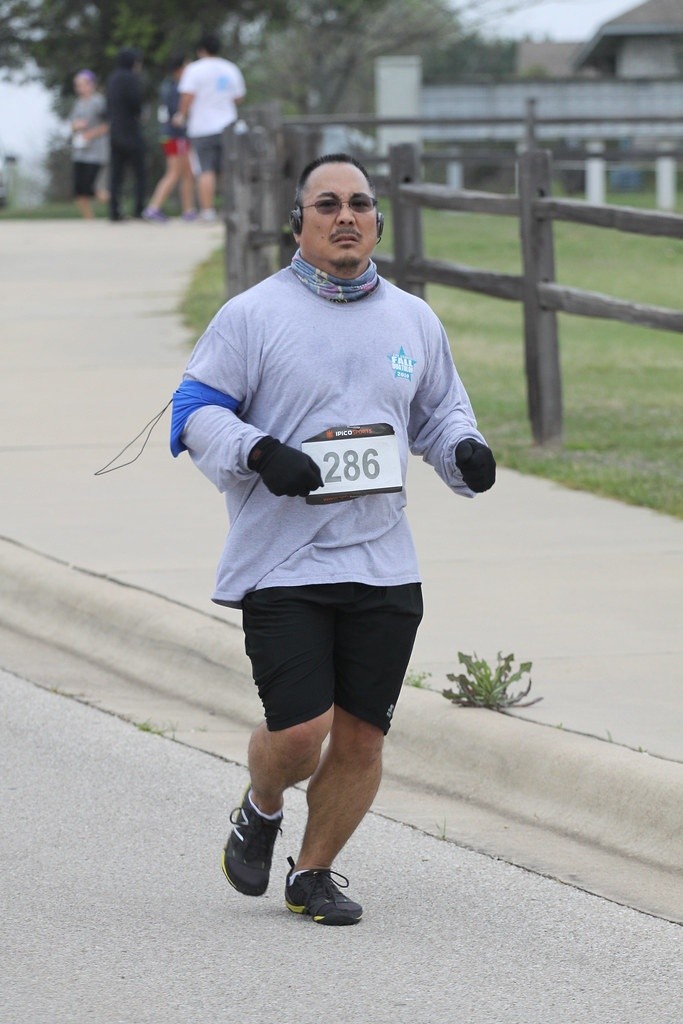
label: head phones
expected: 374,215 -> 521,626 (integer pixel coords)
290,205 -> 384,239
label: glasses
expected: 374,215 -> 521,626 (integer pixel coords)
302,196 -> 377,214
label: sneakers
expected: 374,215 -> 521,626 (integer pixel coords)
223,784 -> 283,896
285,855 -> 363,925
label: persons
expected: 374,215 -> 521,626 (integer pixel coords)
141,31 -> 247,222
104,48 -> 159,221
0,155 -> 17,210
169,154 -> 497,927
62,68 -> 111,220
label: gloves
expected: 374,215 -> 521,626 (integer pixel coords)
455,438 -> 496,493
248,435 -> 324,498
